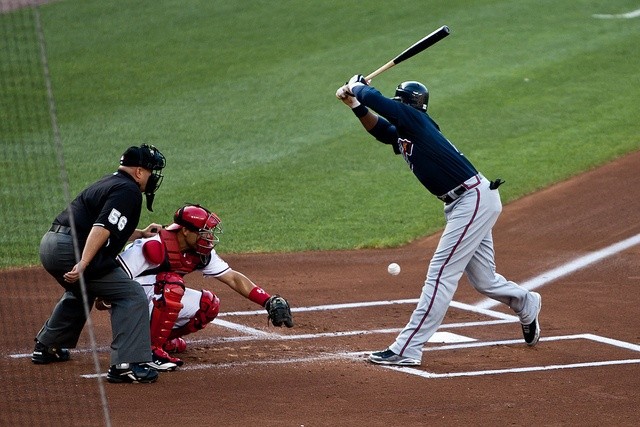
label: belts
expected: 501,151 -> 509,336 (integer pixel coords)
49,225 -> 85,238
439,187 -> 466,205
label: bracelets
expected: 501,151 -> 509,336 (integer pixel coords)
247,286 -> 272,306
352,104 -> 369,118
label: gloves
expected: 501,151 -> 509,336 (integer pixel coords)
490,179 -> 506,191
349,74 -> 368,93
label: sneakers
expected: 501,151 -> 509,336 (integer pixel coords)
367,347 -> 422,366
107,364 -> 157,384
522,292 -> 542,347
31,344 -> 69,363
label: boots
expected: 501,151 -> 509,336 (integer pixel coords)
163,320 -> 186,354
149,349 -> 179,370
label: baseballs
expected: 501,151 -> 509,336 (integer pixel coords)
388,263 -> 401,277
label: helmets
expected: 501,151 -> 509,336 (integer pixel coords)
390,81 -> 429,112
119,144 -> 167,212
165,204 -> 223,258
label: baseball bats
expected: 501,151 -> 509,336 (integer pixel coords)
335,25 -> 450,99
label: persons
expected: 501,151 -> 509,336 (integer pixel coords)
95,202 -> 294,371
32,144 -> 167,384
336,73 -> 543,366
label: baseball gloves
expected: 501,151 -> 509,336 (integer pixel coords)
266,295 -> 292,329
